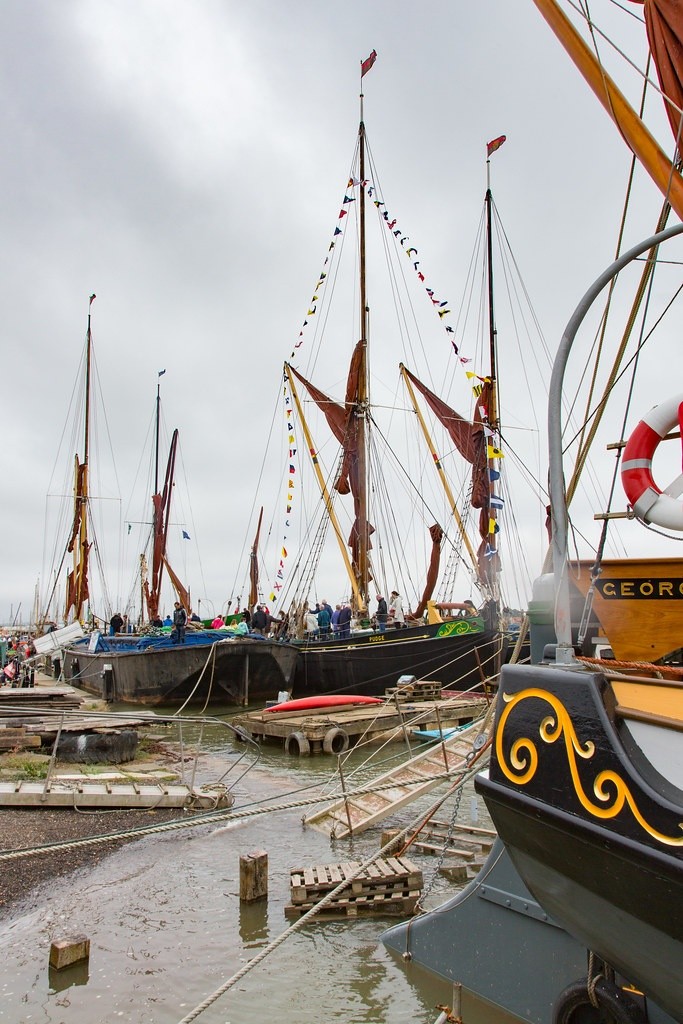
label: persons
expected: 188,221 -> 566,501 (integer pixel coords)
279,610 -> 285,620
192,612 -> 200,622
243,608 -> 251,629
211,615 -> 224,629
238,617 -> 249,633
170,602 -> 187,644
309,600 -> 351,638
111,612 -> 130,633
265,614 -> 284,638
164,615 -> 172,626
252,606 -> 267,636
153,615 -> 163,627
230,618 -> 237,628
390,591 -> 404,629
373,594 -> 388,632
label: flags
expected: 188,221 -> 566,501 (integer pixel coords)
488,135 -> 506,158
90,294 -> 96,305
362,49 -> 377,78
159,369 -> 165,377
361,178 -> 505,556
269,177 -> 361,602
183,530 -> 190,540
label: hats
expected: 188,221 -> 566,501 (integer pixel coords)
257,606 -> 262,609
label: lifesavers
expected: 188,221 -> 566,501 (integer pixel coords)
323,728 -> 350,755
552,976 -> 649,1024
284,732 -> 311,758
620,394 -> 683,530
233,725 -> 253,744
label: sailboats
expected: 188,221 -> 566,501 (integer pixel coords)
383,0 -> 682,1021
29,47 -> 563,714
